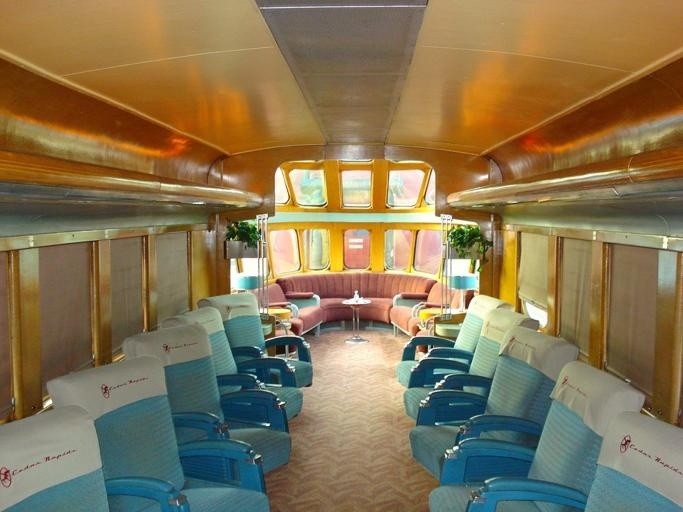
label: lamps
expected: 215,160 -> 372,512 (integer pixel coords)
448,274 -> 477,313
236,274 -> 261,292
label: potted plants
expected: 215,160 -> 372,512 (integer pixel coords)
443,226 -> 493,273
224,222 -> 270,261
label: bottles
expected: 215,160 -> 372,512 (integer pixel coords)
353,291 -> 359,302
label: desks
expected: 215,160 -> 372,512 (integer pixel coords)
340,298 -> 371,345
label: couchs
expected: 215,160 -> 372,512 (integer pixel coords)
241,271 -> 471,357
